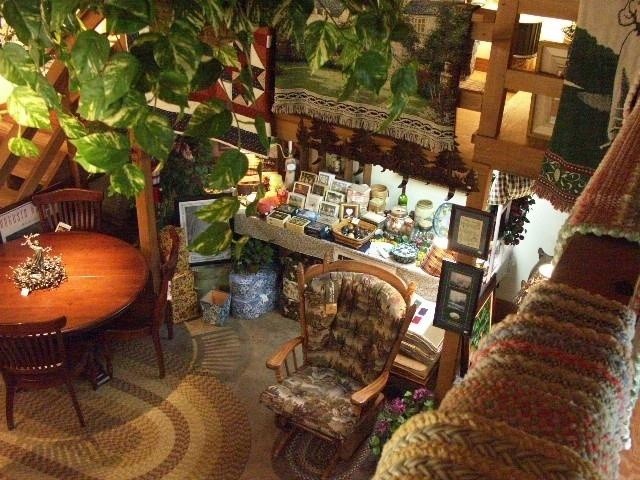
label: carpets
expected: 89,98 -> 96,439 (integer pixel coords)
1,341 -> 253,480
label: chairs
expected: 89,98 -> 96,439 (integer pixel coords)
262,255 -> 416,480
31,187 -> 105,234
98,228 -> 182,379
1,316 -> 86,429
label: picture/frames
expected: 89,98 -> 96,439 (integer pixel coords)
430,202 -> 495,337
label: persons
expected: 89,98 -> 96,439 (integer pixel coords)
27,240 -> 49,271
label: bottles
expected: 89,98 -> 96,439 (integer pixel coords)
384,207 -> 413,238
415,200 -> 434,231
398,187 -> 407,210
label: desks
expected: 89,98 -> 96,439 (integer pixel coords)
3,231 -> 151,391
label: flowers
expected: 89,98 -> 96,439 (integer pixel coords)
5,231 -> 72,292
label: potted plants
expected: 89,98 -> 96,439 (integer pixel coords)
222,235 -> 282,320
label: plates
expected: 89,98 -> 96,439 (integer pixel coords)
434,202 -> 453,239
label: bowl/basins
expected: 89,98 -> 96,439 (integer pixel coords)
391,244 -> 418,264
